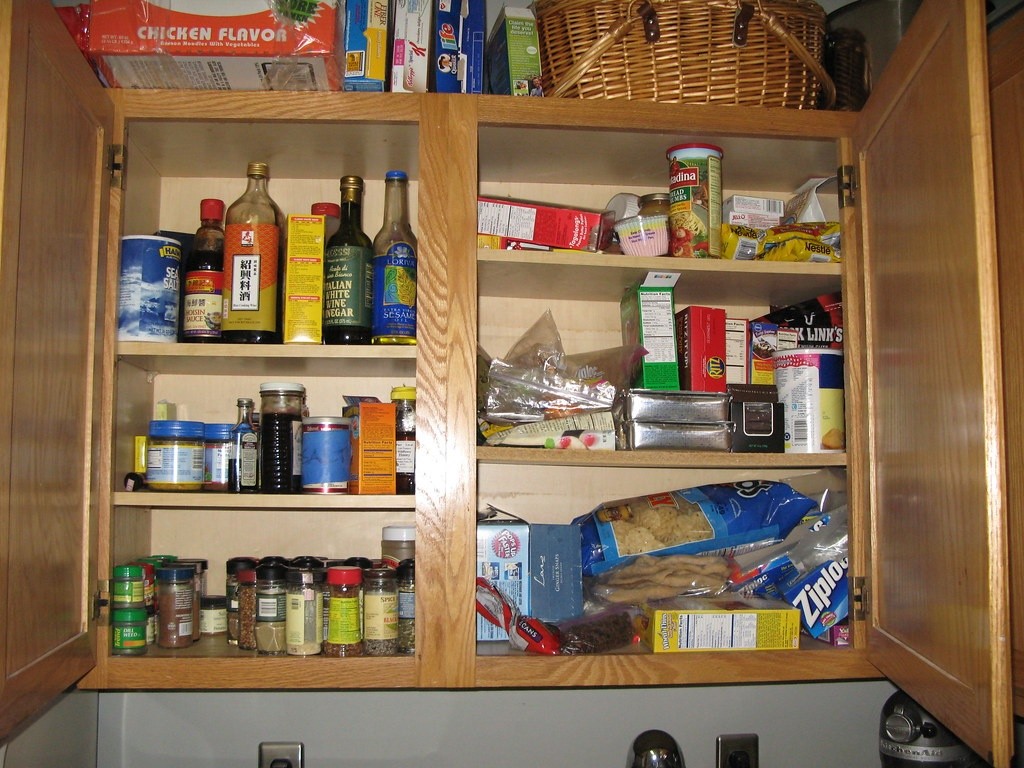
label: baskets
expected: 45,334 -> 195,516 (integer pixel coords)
822,28 -> 871,111
527,0 -> 837,110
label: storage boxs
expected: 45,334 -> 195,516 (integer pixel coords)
54,0 -> 800,655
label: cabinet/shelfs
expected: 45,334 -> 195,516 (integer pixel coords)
0,0 -> 1013,767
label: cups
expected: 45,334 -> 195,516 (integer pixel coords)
616,215 -> 671,257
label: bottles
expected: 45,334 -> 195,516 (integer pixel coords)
114,524 -> 415,655
324,172 -> 373,345
638,193 -> 672,218
390,384 -> 415,496
144,381 -> 350,493
373,166 -> 417,344
307,201 -> 339,245
182,196 -> 224,344
225,159 -> 286,346
